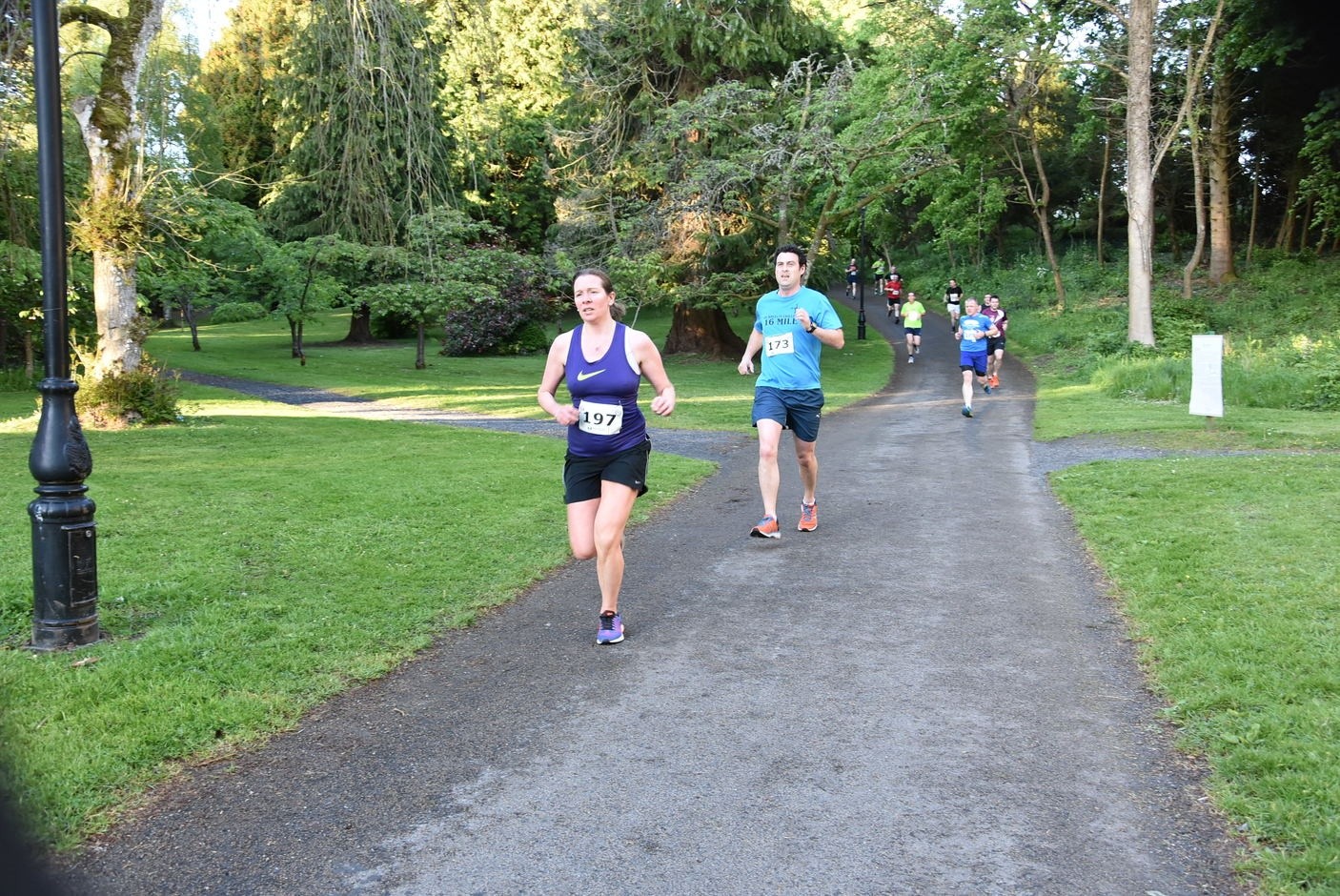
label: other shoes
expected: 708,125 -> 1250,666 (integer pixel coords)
845,291 -> 849,296
950,329 -> 955,334
874,290 -> 884,296
894,318 -> 900,325
852,296 -> 856,300
888,310 -> 893,317
908,355 -> 914,364
913,346 -> 919,354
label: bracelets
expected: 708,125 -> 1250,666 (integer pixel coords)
984,331 -> 986,337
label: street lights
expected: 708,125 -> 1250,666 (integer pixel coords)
854,191 -> 867,339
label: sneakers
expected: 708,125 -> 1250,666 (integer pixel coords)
750,514 -> 781,539
988,376 -> 994,389
961,406 -> 973,418
596,610 -> 625,645
797,497 -> 818,532
991,374 -> 999,386
977,379 -> 992,395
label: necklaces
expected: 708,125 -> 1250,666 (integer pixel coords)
595,346 -> 600,351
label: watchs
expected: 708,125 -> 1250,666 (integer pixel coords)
806,321 -> 816,333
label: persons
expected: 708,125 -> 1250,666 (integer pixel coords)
884,266 -> 904,309
901,293 -> 926,363
737,246 -> 845,537
982,295 -> 1008,389
944,280 -> 963,334
884,275 -> 903,324
955,296 -> 998,418
537,269 -> 675,644
980,293 -> 1001,314
871,258 -> 885,296
845,258 -> 859,300
